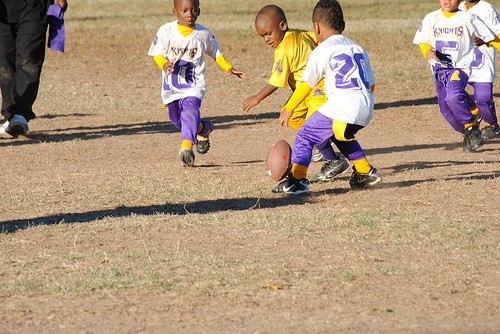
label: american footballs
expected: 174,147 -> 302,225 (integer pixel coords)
267,139 -> 292,181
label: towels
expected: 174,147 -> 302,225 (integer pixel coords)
47,5 -> 68,54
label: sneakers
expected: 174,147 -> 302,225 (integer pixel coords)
179,148 -> 195,167
480,125 -> 500,139
311,141 -> 334,161
0,115 -> 28,139
272,174 -> 308,195
318,158 -> 350,182
349,165 -> 382,188
463,124 -> 484,153
196,120 -> 214,153
475,112 -> 483,122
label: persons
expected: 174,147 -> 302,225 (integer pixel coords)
413,0 -> 500,153
147,0 -> 247,167
0,0 -> 68,140
243,0 -> 384,195
